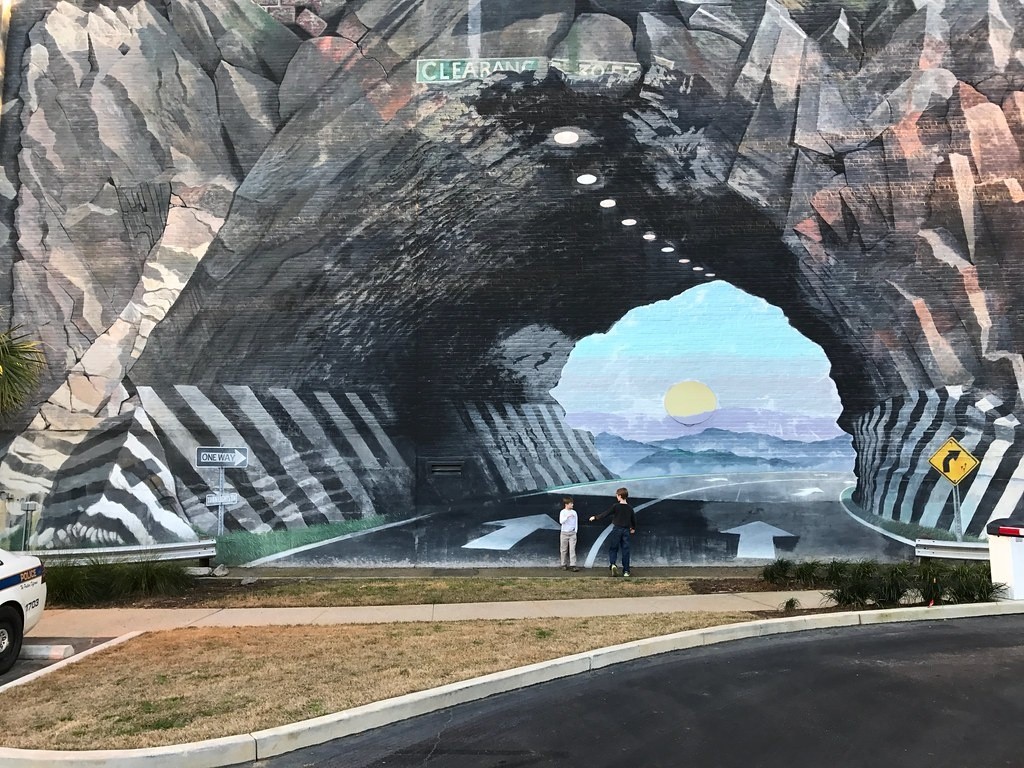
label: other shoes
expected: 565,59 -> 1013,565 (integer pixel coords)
622,571 -> 630,577
570,566 -> 579,572
611,564 -> 619,577
562,565 -> 567,571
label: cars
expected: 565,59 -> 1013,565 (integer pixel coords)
0,542 -> 48,677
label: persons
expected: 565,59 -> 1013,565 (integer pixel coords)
589,488 -> 636,576
560,497 -> 579,571
